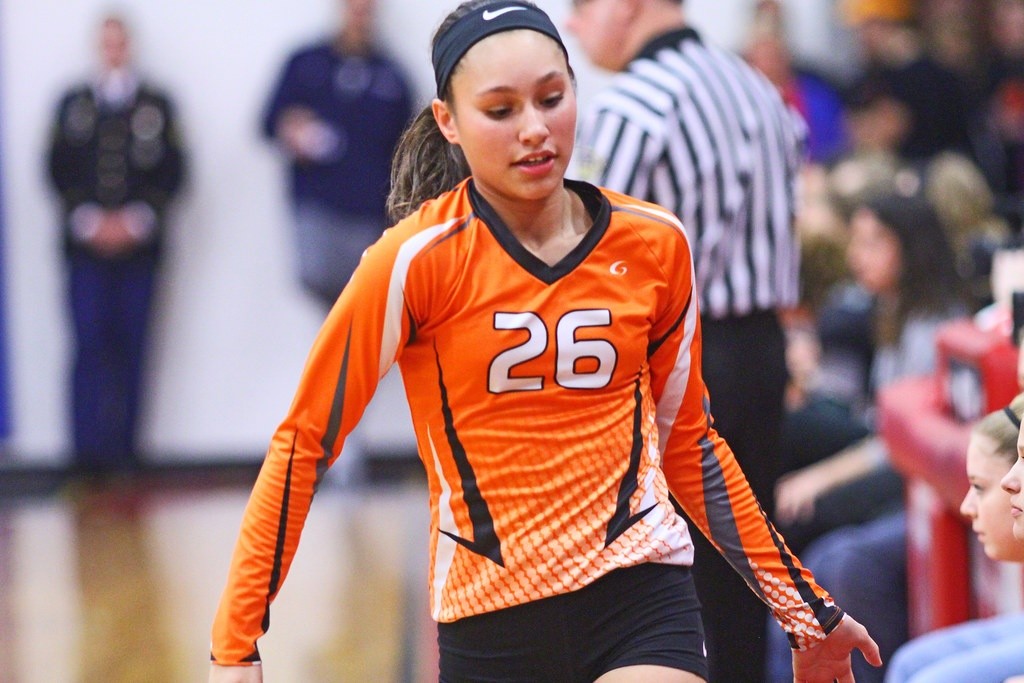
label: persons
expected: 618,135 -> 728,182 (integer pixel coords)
252,0 -> 416,490
563,0 -> 811,683
205,0 -> 884,683
734,0 -> 1024,683
44,15 -> 187,499
